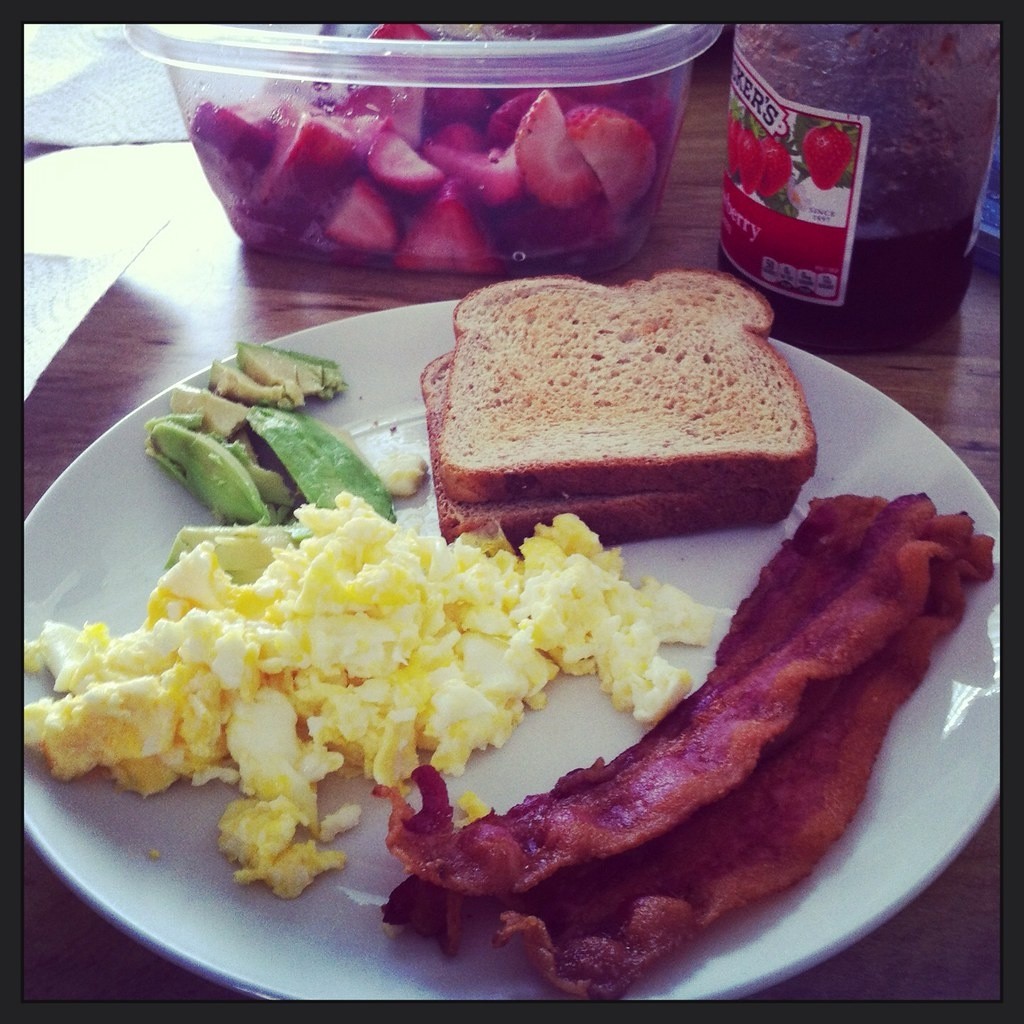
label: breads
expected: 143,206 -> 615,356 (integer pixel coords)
417,265 -> 823,555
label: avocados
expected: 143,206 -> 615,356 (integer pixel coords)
144,338 -> 399,525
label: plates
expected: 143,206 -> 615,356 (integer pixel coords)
22,291 -> 999,1004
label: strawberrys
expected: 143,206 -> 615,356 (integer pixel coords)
188,25 -> 658,281
727,99 -> 852,198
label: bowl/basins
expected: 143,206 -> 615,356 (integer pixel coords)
120,22 -> 726,282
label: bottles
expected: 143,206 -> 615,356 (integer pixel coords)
719,24 -> 999,349
